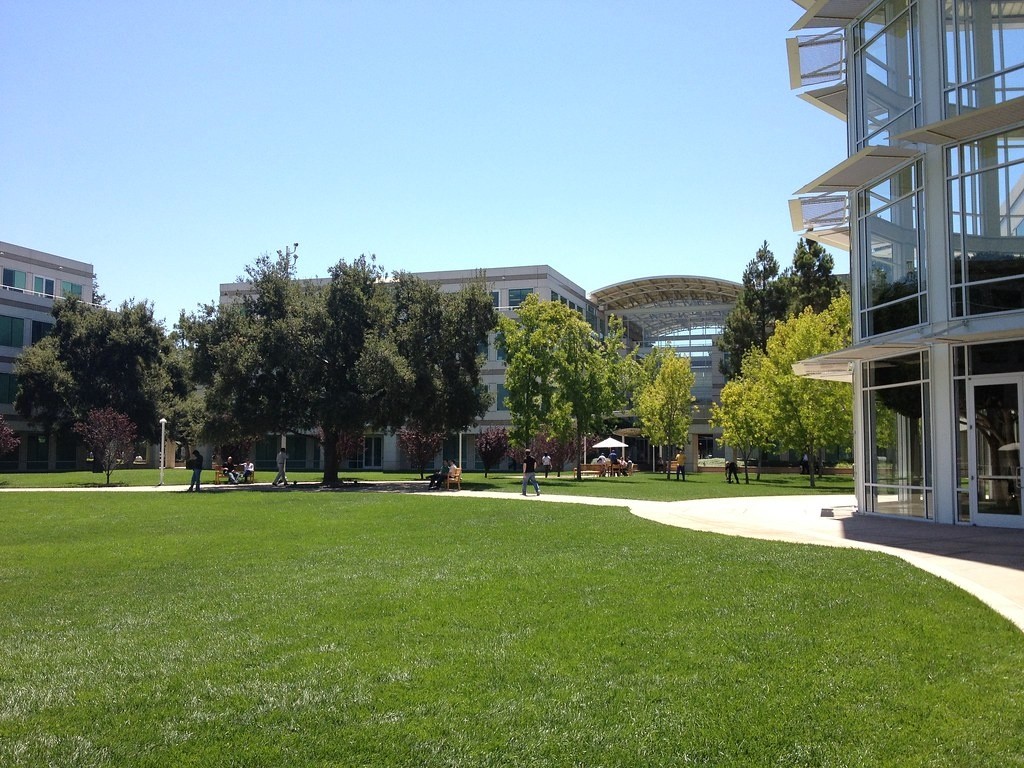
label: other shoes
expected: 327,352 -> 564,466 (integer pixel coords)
187,489 -> 193,492
272,483 -> 277,486
284,484 -> 288,486
195,489 -> 200,492
537,490 -> 540,495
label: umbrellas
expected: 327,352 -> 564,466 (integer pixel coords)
591,435 -> 629,453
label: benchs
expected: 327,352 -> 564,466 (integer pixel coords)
443,468 -> 462,491
215,465 -> 255,485
572,463 -> 640,478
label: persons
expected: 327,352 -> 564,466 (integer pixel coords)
427,458 -> 458,489
658,457 -> 664,474
725,460 -> 739,484
522,448 -> 540,496
595,451 -> 633,477
273,448 -> 290,486
187,450 -> 204,491
542,451 -> 552,478
675,450 -> 687,481
222,456 -> 239,484
211,454 -> 223,472
239,459 -> 255,482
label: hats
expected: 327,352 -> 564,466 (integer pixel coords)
726,461 -> 729,464
